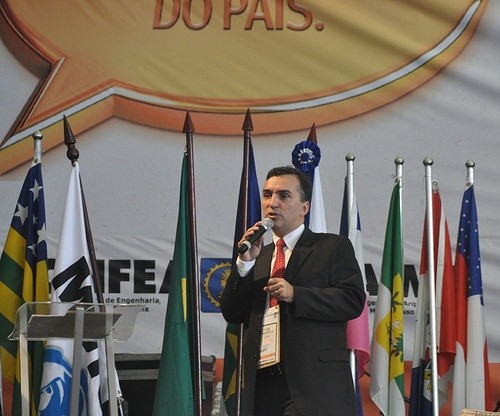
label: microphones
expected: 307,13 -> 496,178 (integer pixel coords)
238,217 -> 274,255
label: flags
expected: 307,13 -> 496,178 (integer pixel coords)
0,161 -> 49,416
150,152 -> 207,416
364,186 -> 404,416
452,185 -> 490,416
302,165 -> 326,233
339,174 -> 370,377
37,163 -> 125,416
222,135 -> 261,416
409,190 -> 456,416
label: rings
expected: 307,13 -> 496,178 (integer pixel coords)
275,290 -> 279,294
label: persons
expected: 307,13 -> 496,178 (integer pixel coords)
220,167 -> 366,416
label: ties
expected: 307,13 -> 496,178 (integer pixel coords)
270,239 -> 286,306
